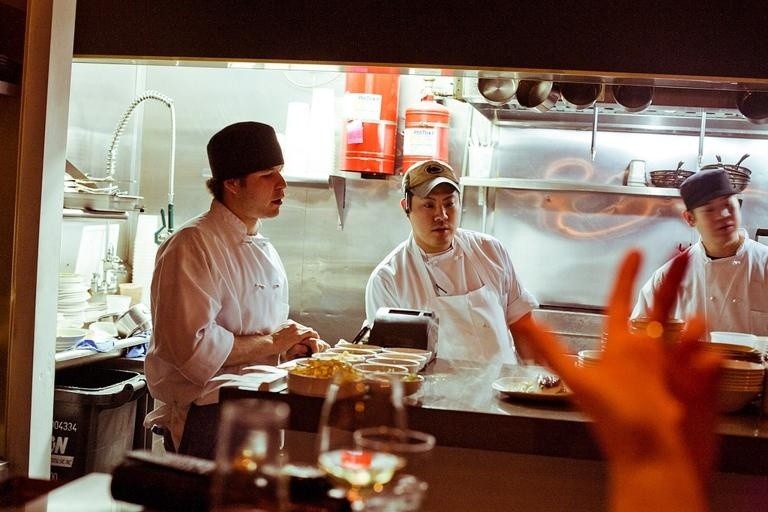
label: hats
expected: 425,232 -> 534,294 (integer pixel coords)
207,122 -> 284,182
680,168 -> 740,211
402,160 -> 461,199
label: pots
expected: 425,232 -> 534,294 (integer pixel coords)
560,78 -> 602,109
734,90 -> 768,125
611,79 -> 655,112
477,71 -> 519,106
516,75 -> 560,113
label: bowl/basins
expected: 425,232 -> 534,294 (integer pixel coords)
311,343 -> 433,379
577,317 -> 768,414
106,295 -> 131,314
117,303 -> 153,339
56,329 -> 90,353
119,283 -> 143,303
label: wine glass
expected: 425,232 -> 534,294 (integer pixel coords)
317,379 -> 411,512
355,428 -> 435,511
215,401 -> 288,511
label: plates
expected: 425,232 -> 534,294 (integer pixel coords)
57,272 -> 89,328
287,370 -> 362,400
491,377 -> 576,399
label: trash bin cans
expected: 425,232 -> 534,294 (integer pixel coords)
50,365 -> 147,483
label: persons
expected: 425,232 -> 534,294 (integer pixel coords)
363,159 -> 547,367
513,242 -> 728,512
139,120 -> 331,450
629,167 -> 768,336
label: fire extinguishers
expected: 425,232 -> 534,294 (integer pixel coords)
402,90 -> 455,175
339,66 -> 399,175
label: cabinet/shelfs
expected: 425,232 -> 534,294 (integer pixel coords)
54,210 -> 129,374
457,67 -> 768,373
